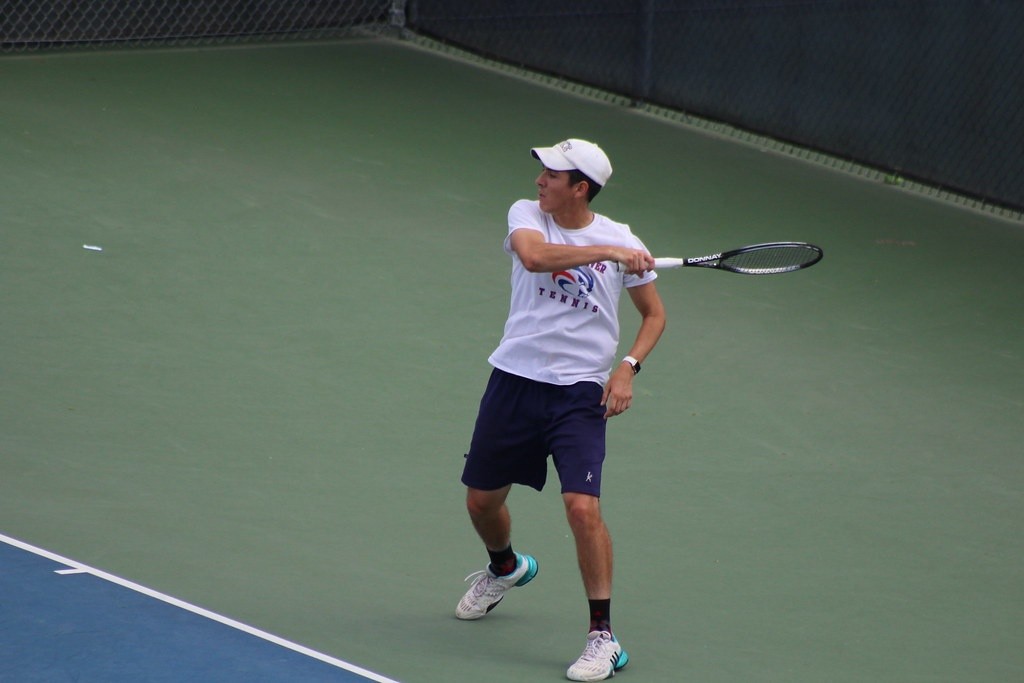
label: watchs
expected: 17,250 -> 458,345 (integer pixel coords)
622,356 -> 641,375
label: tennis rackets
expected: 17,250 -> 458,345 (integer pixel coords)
616,241 -> 824,275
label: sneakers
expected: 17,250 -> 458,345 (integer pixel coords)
455,550 -> 538,619
566,630 -> 628,682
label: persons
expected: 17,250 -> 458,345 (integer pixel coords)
456,137 -> 667,683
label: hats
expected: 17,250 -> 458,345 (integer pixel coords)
530,139 -> 612,187
